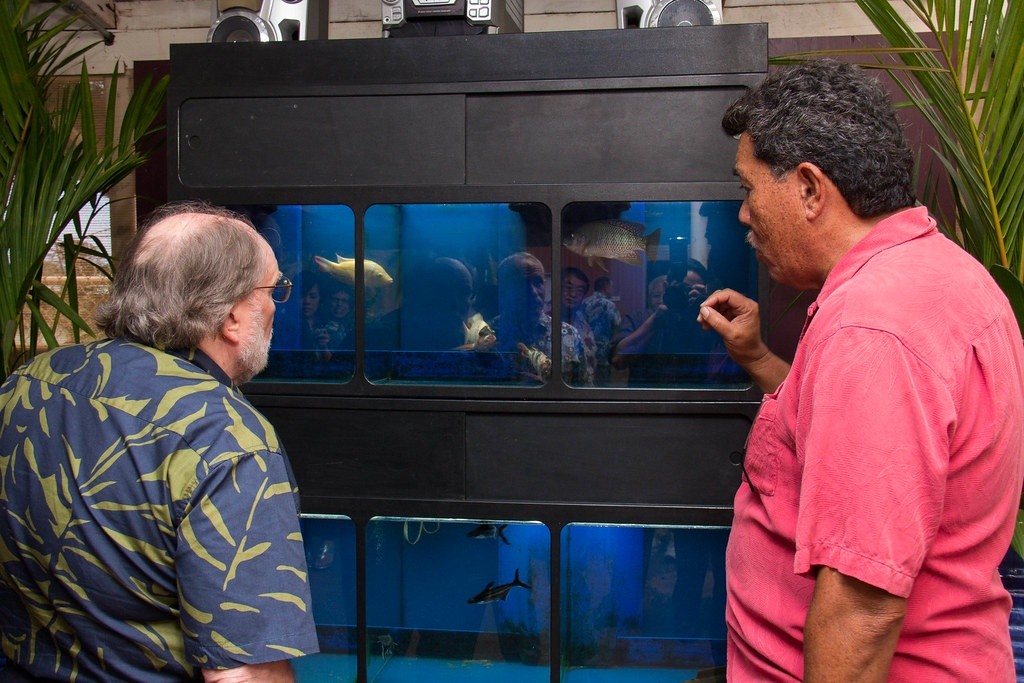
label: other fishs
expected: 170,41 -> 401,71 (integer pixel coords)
465,567 -> 533,607
515,341 -> 553,384
465,521 -> 511,546
452,304 -> 502,352
314,252 -> 393,297
559,215 -> 661,272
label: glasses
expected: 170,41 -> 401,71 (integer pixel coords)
251,275 -> 293,304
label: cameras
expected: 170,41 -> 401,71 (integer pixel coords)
662,236 -> 696,311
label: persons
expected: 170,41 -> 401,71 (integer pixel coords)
0,212 -> 319,683
606,258 -> 707,389
580,277 -> 621,389
606,274 -> 666,388
542,268 -> 597,388
695,61 -> 1024,683
267,252 -> 596,388
